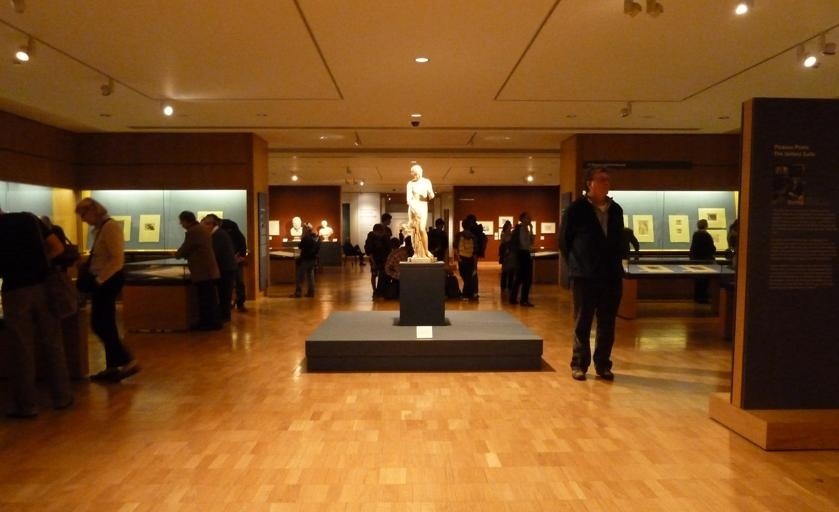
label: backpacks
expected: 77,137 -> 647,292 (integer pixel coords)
457,236 -> 475,258
511,224 -> 521,250
386,248 -> 408,279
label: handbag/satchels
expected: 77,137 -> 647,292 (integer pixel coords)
621,226 -> 640,260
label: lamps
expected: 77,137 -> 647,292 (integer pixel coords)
817,32 -> 839,56
645,1 -> 665,20
616,100 -> 636,119
734,1 -> 754,17
797,43 -> 817,69
0,1 -> 177,119
622,0 -> 643,18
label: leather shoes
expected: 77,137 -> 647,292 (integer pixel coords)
571,365 -> 586,380
596,369 -> 614,380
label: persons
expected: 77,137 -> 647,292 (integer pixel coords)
405,163 -> 435,261
289,222 -> 321,298
497,208 -> 535,306
623,229 -> 638,256
726,216 -> 740,272
290,216 -> 303,236
319,220 -> 333,238
365,214 -> 487,299
688,219 -> 717,303
555,161 -> 630,381
175,210 -> 251,333
1,196 -> 145,420
345,237 -> 366,266
